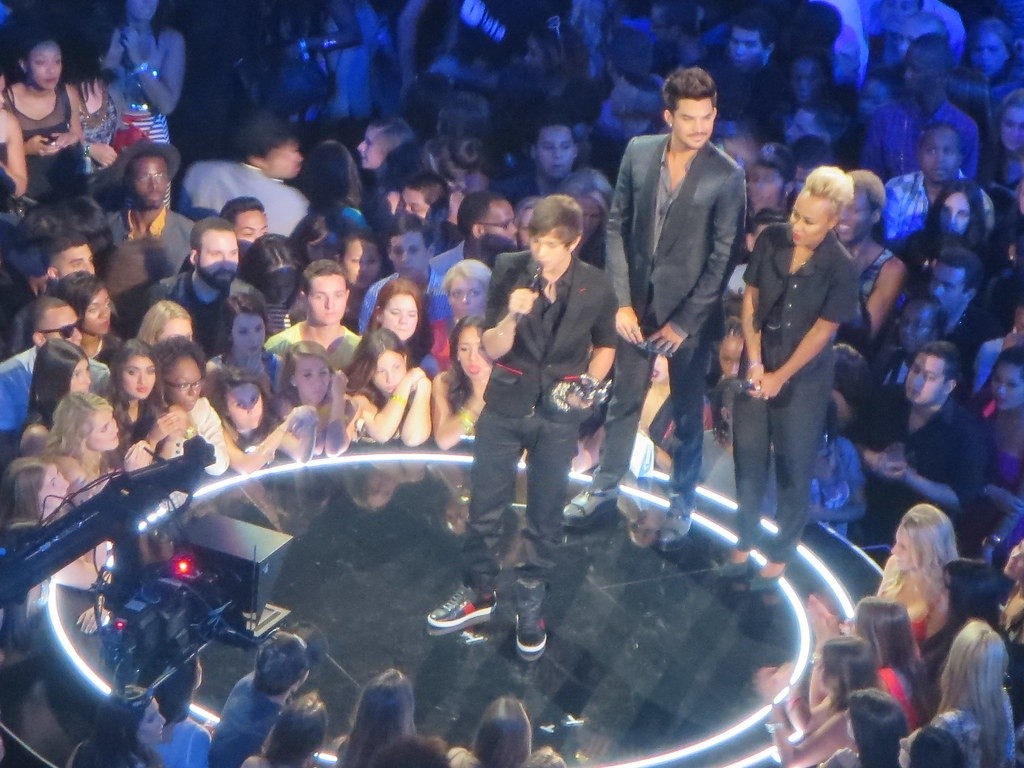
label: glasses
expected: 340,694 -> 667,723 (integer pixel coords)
481,217 -> 515,230
134,173 -> 173,186
392,246 -> 425,254
162,379 -> 206,392
902,318 -> 933,331
548,15 -> 561,41
39,319 -> 82,338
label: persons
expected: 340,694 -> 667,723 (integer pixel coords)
0,0 -> 1024,768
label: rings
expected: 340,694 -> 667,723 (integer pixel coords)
631,327 -> 640,335
763,397 -> 769,401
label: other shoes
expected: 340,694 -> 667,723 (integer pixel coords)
720,549 -> 752,577
563,484 -> 619,520
750,557 -> 790,592
655,495 -> 695,553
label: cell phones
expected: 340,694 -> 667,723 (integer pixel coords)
808,595 -> 832,622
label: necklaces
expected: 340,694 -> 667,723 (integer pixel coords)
792,247 -> 814,268
548,258 -> 571,294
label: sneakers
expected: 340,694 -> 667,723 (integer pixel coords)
427,584 -> 497,628
515,602 -> 547,653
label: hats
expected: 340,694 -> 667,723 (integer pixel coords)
255,621 -> 329,681
110,142 -> 180,193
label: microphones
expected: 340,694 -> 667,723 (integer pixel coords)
515,261 -> 544,323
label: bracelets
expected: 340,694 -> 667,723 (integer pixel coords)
496,322 -> 516,337
127,62 -> 148,77
456,406 -> 479,433
297,38 -> 308,54
276,426 -> 287,434
82,140 -> 91,157
811,652 -> 824,664
390,395 -> 408,406
747,360 -> 763,369
184,424 -> 199,440
141,438 -> 155,450
765,723 -> 788,733
322,36 -> 337,52
786,696 -> 801,714
255,445 -> 274,461
